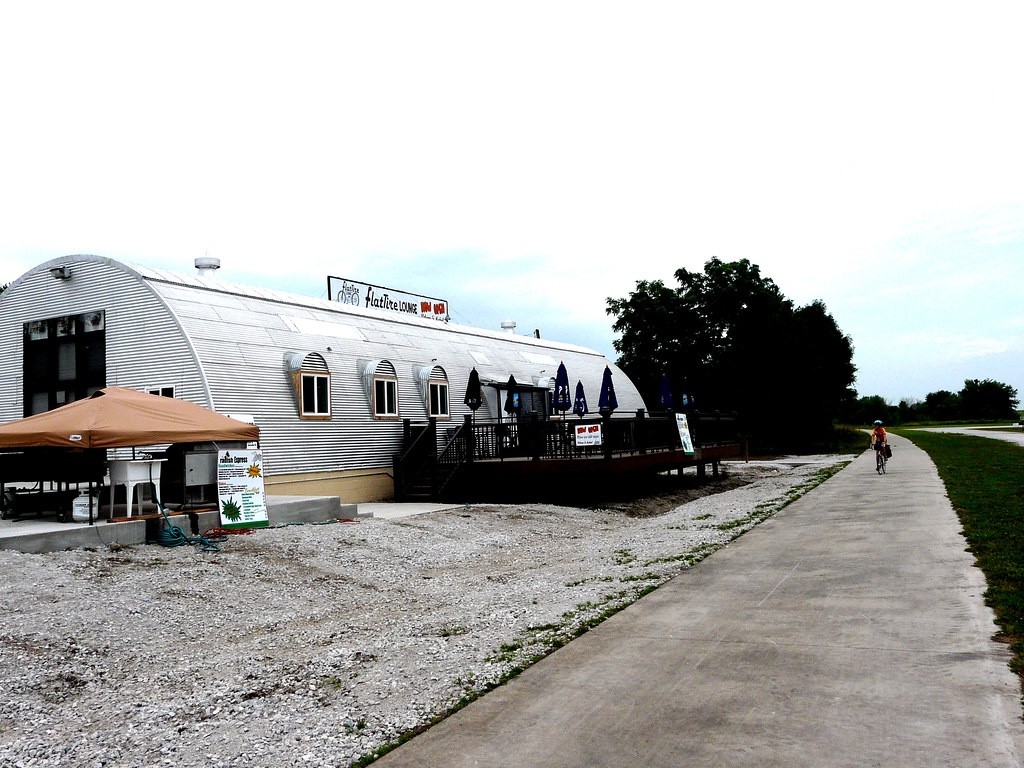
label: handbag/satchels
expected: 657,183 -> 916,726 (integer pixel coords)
884,444 -> 892,458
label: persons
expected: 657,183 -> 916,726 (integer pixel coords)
870,420 -> 889,471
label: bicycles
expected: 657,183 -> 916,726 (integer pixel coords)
871,444 -> 886,475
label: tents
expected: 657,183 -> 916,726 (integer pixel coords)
1,384 -> 260,526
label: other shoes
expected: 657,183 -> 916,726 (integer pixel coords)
885,458 -> 888,461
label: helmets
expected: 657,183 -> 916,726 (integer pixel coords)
874,420 -> 883,425
